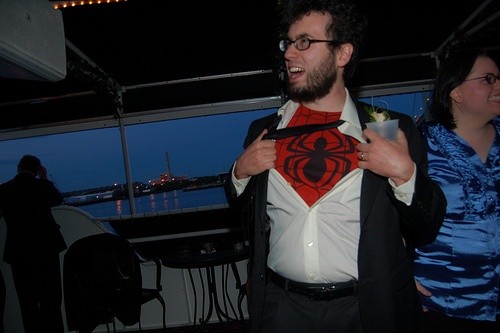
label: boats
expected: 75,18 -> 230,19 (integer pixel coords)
180,172 -> 230,191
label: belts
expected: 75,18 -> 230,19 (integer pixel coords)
267,269 -> 359,301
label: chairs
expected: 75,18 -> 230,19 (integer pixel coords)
50,205 -> 167,333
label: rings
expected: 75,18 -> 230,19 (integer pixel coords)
361,152 -> 364,160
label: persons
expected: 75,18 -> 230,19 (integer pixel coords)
222,0 -> 448,333
399,44 -> 500,333
0,153 -> 67,333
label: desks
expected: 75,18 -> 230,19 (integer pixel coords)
127,228 -> 250,330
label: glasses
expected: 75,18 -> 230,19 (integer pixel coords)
279,37 -> 337,52
464,73 -> 500,84
